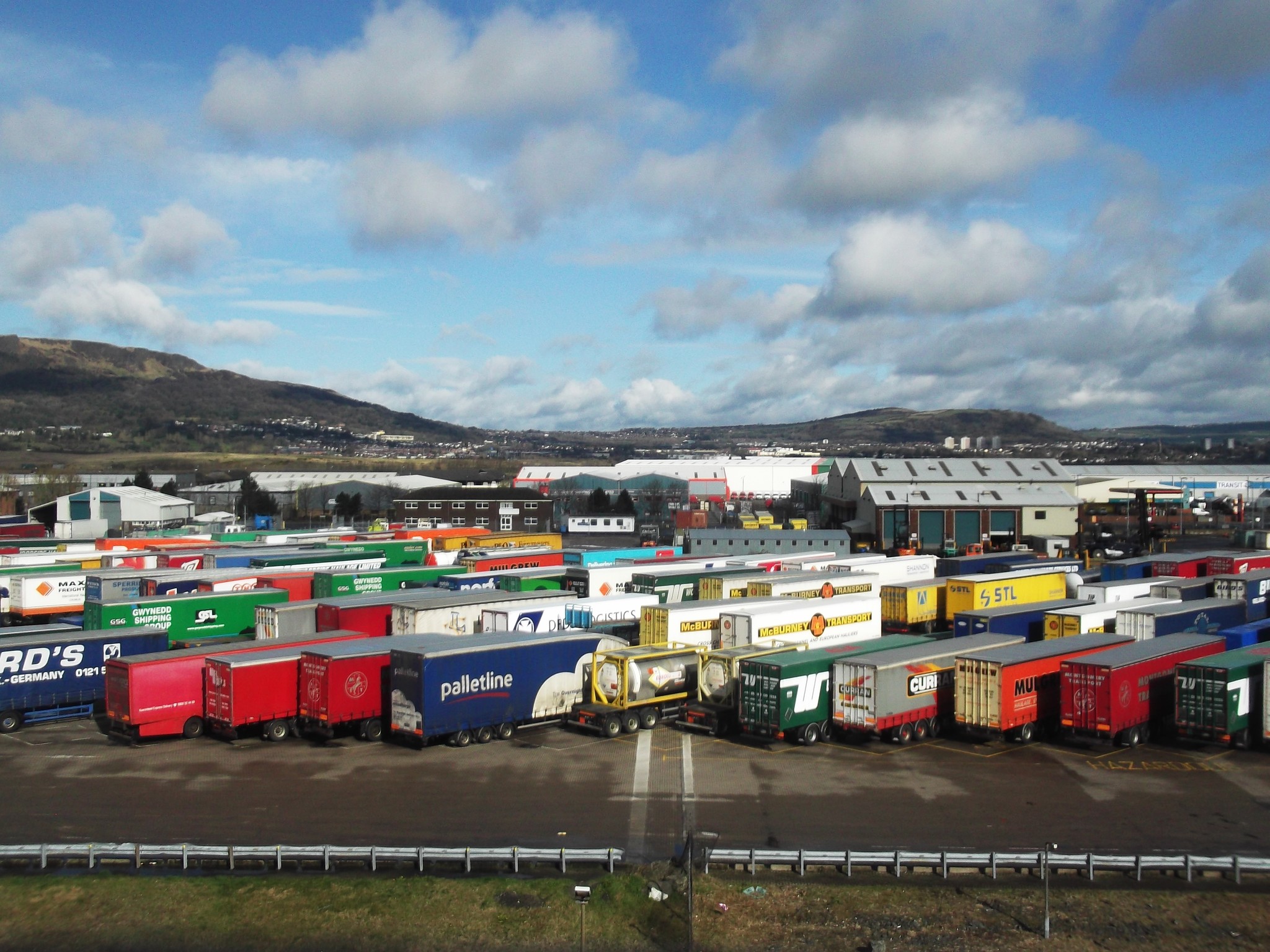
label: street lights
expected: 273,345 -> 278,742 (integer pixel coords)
1127,479 -> 1135,529
1180,477 -> 1187,535
